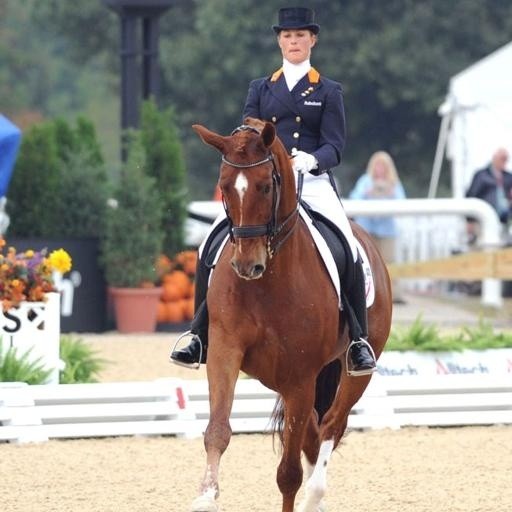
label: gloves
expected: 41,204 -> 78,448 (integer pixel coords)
291,148 -> 318,173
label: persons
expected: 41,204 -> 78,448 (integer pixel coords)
169,6 -> 375,371
464,147 -> 512,249
349,149 -> 410,306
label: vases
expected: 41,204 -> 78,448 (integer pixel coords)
1,292 -> 60,387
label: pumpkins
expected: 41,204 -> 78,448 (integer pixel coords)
139,250 -> 197,322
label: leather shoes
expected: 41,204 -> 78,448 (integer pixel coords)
169,333 -> 208,365
349,337 -> 377,371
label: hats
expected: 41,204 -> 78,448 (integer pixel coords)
270,6 -> 320,34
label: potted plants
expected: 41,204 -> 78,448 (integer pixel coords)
98,128 -> 164,332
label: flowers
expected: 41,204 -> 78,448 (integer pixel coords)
0,236 -> 72,301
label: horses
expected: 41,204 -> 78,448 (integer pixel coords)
189,122 -> 394,511
